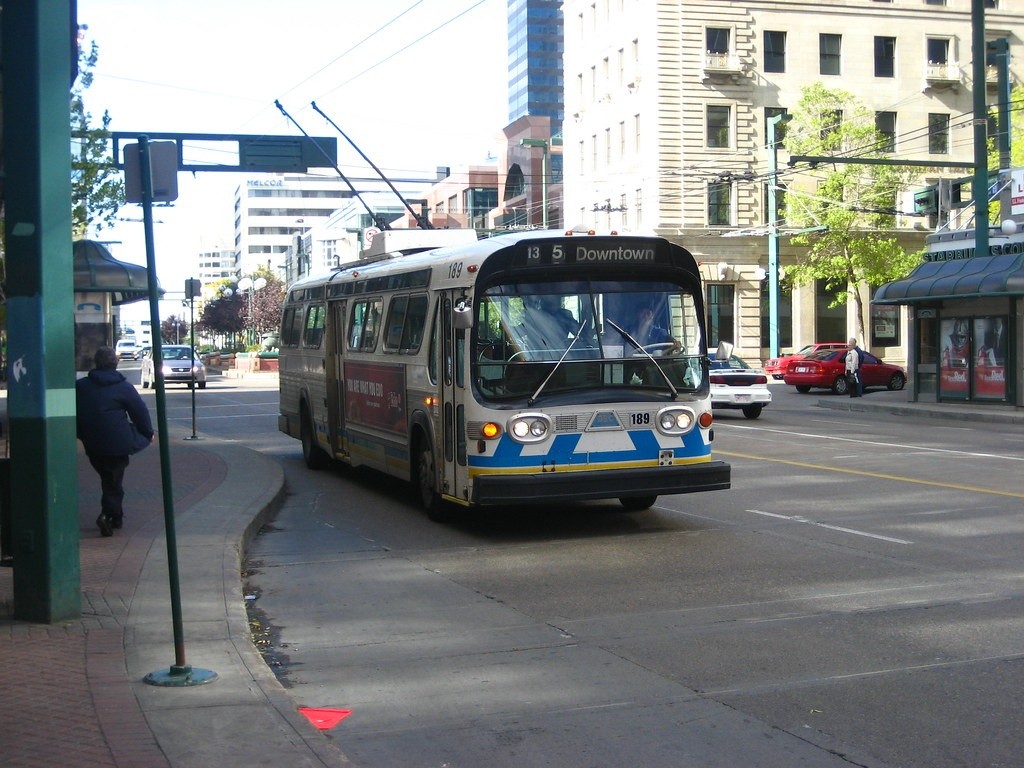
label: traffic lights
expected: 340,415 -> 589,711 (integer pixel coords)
914,191 -> 935,209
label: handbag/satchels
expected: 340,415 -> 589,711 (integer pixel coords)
849,375 -> 856,384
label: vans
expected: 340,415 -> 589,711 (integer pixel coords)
142,346 -> 151,358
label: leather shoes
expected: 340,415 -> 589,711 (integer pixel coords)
96,513 -> 123,537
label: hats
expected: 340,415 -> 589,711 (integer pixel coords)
637,302 -> 653,312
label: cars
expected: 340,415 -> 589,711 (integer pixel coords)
140,345 -> 206,389
683,348 -> 773,418
765,344 -> 849,380
783,348 -> 907,395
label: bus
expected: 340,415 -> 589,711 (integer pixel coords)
277,227 -> 734,521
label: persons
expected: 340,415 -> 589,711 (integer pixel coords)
76,347 -> 154,537
499,294 -> 600,355
176,348 -> 191,360
845,338 -> 863,399
986,316 -> 1006,365
618,306 -> 681,354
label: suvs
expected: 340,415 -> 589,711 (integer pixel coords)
116,339 -> 137,360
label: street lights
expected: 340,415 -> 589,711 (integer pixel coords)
520,137 -> 548,229
